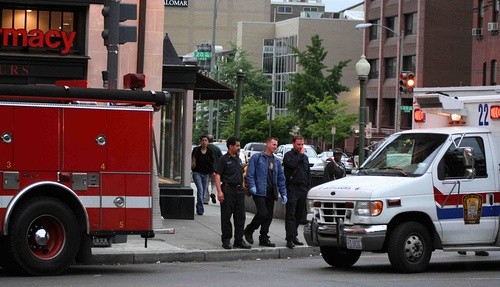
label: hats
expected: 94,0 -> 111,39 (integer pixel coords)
333,148 -> 344,154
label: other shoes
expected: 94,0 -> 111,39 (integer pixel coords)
475,251 -> 489,256
457,250 -> 466,255
210,194 -> 216,204
202,202 -> 209,205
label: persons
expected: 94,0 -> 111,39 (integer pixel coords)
352,142 -> 360,171
243,136 -> 289,247
323,148 -> 347,184
203,133 -> 222,204
282,135 -> 313,248
212,137 -> 251,249
457,250 -> 490,257
364,145 -> 372,160
191,135 -> 216,215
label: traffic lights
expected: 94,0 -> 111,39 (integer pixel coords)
400,71 -> 415,94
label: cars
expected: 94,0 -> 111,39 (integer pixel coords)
193,140 -> 354,176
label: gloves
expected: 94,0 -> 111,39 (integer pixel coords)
251,187 -> 257,196
281,196 -> 288,204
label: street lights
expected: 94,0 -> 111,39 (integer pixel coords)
355,55 -> 371,167
355,24 -> 406,133
233,69 -> 246,142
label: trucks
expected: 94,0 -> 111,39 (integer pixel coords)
304,85 -> 500,275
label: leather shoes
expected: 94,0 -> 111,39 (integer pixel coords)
285,242 -> 295,247
244,230 -> 253,244
292,239 -> 304,245
233,239 -> 252,249
259,237 -> 276,246
222,241 -> 233,249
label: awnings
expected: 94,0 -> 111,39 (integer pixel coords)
193,74 -> 235,102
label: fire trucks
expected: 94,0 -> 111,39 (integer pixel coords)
0,73 -> 176,276
